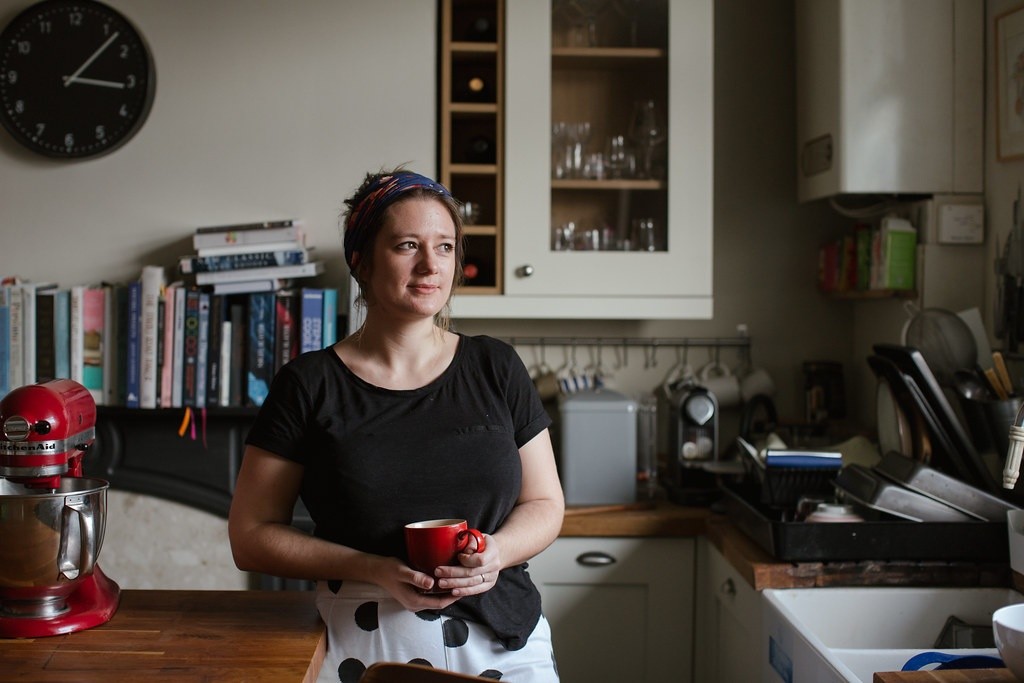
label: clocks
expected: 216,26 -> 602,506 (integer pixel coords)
0,0 -> 157,160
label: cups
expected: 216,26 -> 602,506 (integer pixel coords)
529,365 -> 558,399
552,216 -> 667,252
698,364 -> 741,407
557,361 -> 593,395
454,201 -> 480,225
403,518 -> 487,596
551,103 -> 665,180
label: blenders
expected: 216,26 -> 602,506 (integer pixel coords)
0,379 -> 121,635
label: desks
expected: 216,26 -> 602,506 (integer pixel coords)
0,590 -> 328,683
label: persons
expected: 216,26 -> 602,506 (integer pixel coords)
227,165 -> 566,683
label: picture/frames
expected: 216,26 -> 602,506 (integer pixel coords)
993,5 -> 1024,164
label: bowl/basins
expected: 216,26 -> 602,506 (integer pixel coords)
990,603 -> 1024,683
803,505 -> 868,522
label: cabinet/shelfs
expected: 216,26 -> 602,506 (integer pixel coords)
504,0 -> 714,299
434,1 -> 505,296
525,537 -> 693,683
695,538 -> 762,683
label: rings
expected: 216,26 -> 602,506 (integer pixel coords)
480,574 -> 486,583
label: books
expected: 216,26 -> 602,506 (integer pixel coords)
0,215 -> 368,408
